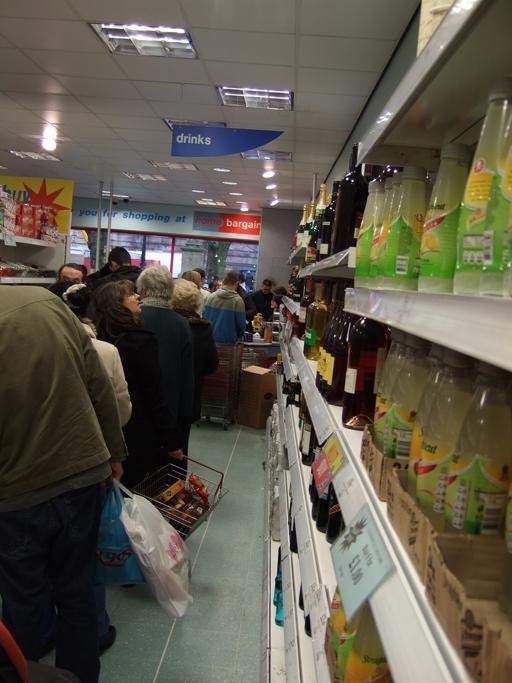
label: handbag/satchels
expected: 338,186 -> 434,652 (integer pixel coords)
97,472 -> 193,622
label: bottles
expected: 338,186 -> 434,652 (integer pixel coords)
292,87 -> 512,298
278,281 -> 509,537
268,553 -> 394,683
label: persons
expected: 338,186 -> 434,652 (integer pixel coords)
200,273 -> 289,341
1,285 -> 128,682
38,246 -> 219,659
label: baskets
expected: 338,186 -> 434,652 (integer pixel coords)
196,335 -> 242,431
134,463 -> 227,540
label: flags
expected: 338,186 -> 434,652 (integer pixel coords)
1,174 -> 75,234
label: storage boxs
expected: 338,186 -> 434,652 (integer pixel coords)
239,365 -> 272,428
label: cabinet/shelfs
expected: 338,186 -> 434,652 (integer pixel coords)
0,233 -> 72,290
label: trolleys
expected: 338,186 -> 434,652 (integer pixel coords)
194,336 -> 241,431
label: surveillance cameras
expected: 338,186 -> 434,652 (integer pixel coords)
124,199 -> 128,202
112,199 -> 119,205
